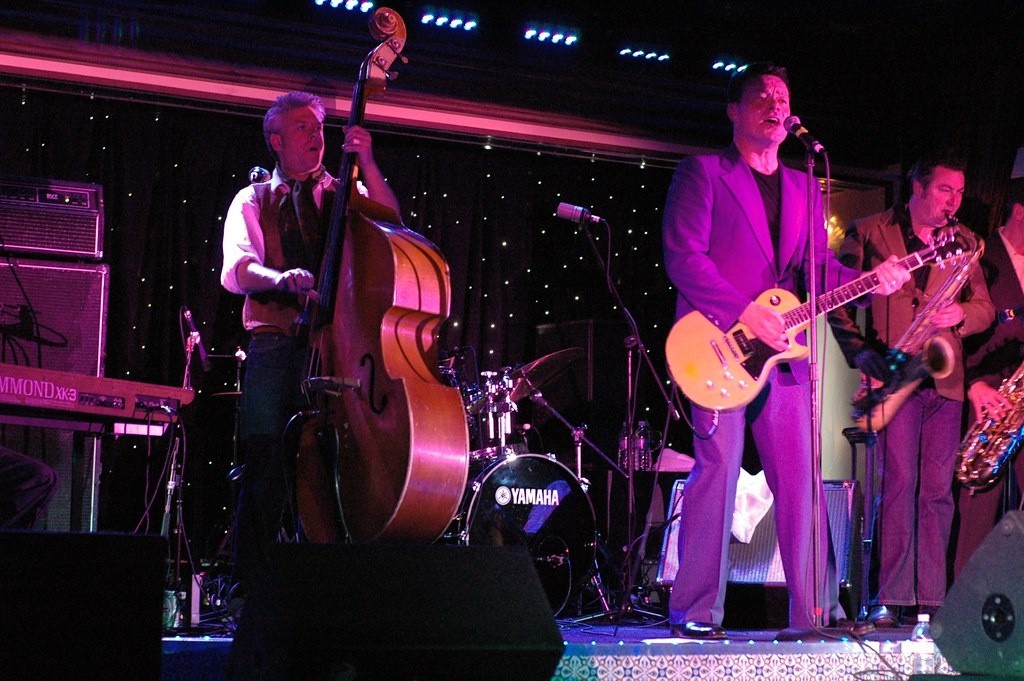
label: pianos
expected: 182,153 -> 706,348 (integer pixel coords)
0,361 -> 195,536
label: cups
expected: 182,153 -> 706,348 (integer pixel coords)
648,431 -> 662,451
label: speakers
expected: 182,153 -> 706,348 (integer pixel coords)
182,355 -> 249,567
0,255 -> 110,532
224,542 -> 565,681
928,508 -> 1024,678
534,317 -> 647,588
655,479 -> 872,631
0,529 -> 169,681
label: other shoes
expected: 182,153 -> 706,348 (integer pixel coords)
873,605 -> 898,627
918,606 -> 938,624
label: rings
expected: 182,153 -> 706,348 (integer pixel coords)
888,280 -> 896,287
353,137 -> 360,146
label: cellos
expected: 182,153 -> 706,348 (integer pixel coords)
295,7 -> 470,546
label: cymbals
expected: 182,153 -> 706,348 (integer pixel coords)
466,346 -> 585,414
212,391 -> 242,398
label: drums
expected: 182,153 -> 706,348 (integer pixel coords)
439,366 -> 477,439
444,454 -> 597,610
464,443 -> 531,487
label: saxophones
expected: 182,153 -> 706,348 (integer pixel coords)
955,360 -> 1024,490
852,212 -> 986,434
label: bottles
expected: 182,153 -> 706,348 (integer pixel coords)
912,613 -> 931,641
633,421 -> 651,470
618,422 -> 628,471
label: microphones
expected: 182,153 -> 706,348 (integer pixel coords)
535,557 -> 569,569
783,115 -> 826,155
999,305 -> 1024,324
556,202 -> 606,224
185,306 -> 212,371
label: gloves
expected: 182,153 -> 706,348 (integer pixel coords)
853,347 -> 889,379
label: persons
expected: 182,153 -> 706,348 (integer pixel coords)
827,150 -> 996,630
953,186 -> 1024,588
663,63 -> 912,642
219,91 -> 404,617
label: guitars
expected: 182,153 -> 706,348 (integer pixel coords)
665,230 -> 974,411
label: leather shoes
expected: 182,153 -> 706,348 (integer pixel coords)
673,620 -> 728,638
830,618 -> 876,636
